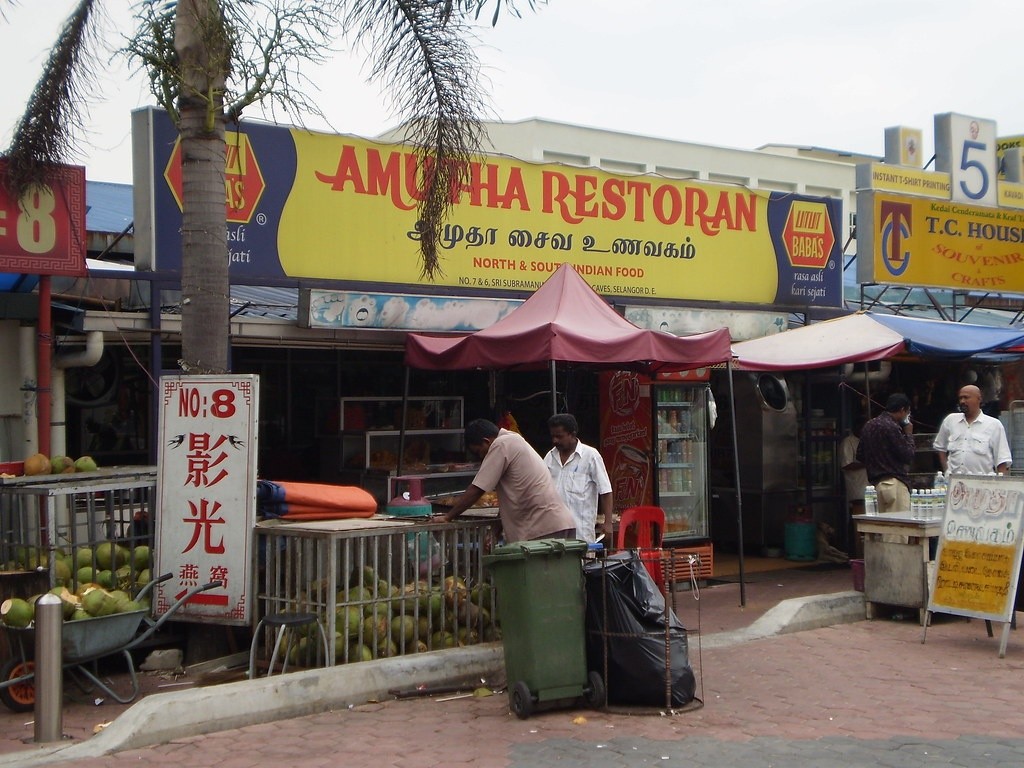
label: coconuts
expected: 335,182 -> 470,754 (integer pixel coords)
0,453 -> 155,628
269,561 -> 503,671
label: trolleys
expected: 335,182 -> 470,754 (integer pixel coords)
1,572 -> 220,714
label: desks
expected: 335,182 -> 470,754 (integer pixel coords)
851,509 -> 947,627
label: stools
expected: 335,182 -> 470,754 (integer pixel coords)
249,612 -> 330,679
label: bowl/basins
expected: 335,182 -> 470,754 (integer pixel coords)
452,461 -> 473,471
427,463 -> 449,473
0,461 -> 25,477
810,408 -> 824,417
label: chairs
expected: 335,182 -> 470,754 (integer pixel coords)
609,505 -> 667,599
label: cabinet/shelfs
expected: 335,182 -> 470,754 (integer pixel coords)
902,432 -> 948,489
339,396 -> 482,509
795,417 -> 838,490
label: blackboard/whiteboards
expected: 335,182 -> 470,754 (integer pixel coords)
928,473 -> 1024,623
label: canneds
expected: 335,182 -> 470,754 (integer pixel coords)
656,387 -> 694,493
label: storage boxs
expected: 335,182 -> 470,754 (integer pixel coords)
849,558 -> 865,593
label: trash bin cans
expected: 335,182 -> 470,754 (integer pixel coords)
481,536 -> 604,720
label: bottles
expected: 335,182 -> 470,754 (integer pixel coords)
934,471 -> 944,488
661,507 -> 694,532
798,421 -> 832,440
956,470 -> 1003,477
945,469 -> 952,487
659,469 -> 696,492
656,387 -> 690,401
910,488 -> 947,522
658,409 -> 689,434
659,439 -> 695,463
864,485 -> 880,517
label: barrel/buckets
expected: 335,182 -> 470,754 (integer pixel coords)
846,557 -> 866,591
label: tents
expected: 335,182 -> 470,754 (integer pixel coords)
406,259 -> 1024,630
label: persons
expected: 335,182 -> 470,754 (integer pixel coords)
932,385 -> 1013,476
837,415 -> 873,560
426,413 -> 613,567
855,393 -> 916,560
